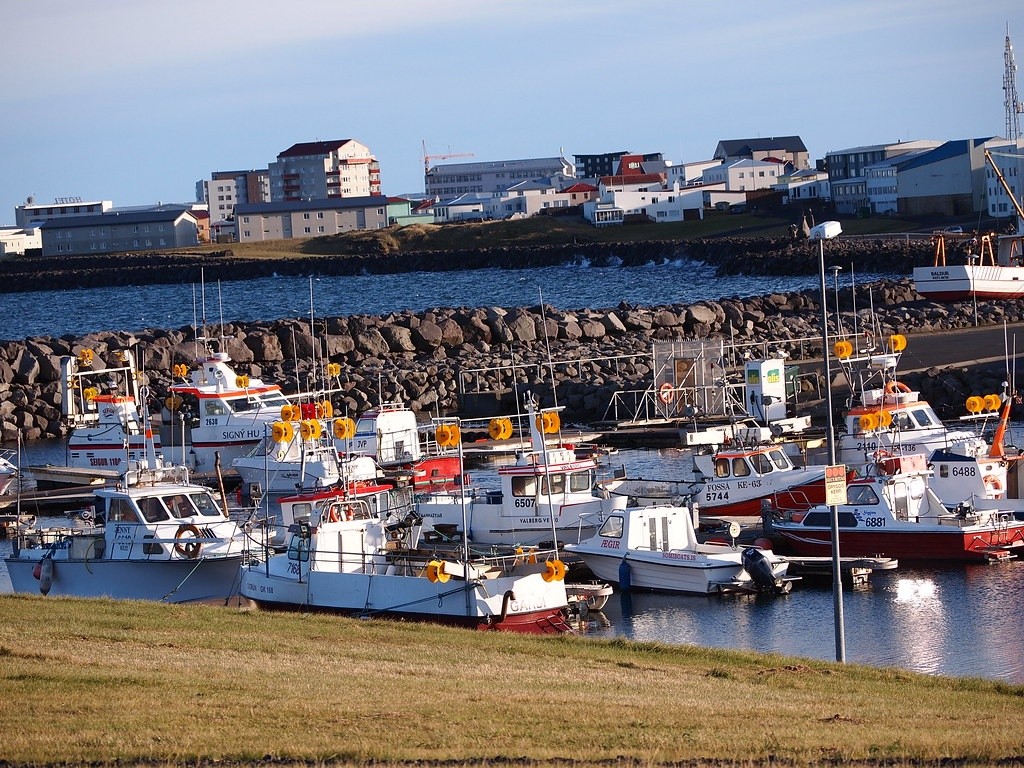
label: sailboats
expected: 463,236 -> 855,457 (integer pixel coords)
913,148 -> 1024,300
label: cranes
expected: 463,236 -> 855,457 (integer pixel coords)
419,140 -> 476,174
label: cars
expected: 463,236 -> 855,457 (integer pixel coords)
944,226 -> 962,233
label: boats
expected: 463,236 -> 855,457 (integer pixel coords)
595,262 -> 1024,524
238,406 -> 572,635
3,349 -> 278,603
0,448 -> 18,495
771,336 -> 1024,566
563,504 -> 802,597
62,266 -> 629,560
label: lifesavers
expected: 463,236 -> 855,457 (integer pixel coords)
174,525 -> 201,559
659,382 -> 674,405
886,381 -> 911,394
329,500 -> 354,523
873,450 -> 895,459
983,474 -> 1003,499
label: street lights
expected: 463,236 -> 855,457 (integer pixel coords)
810,219 -> 846,667
